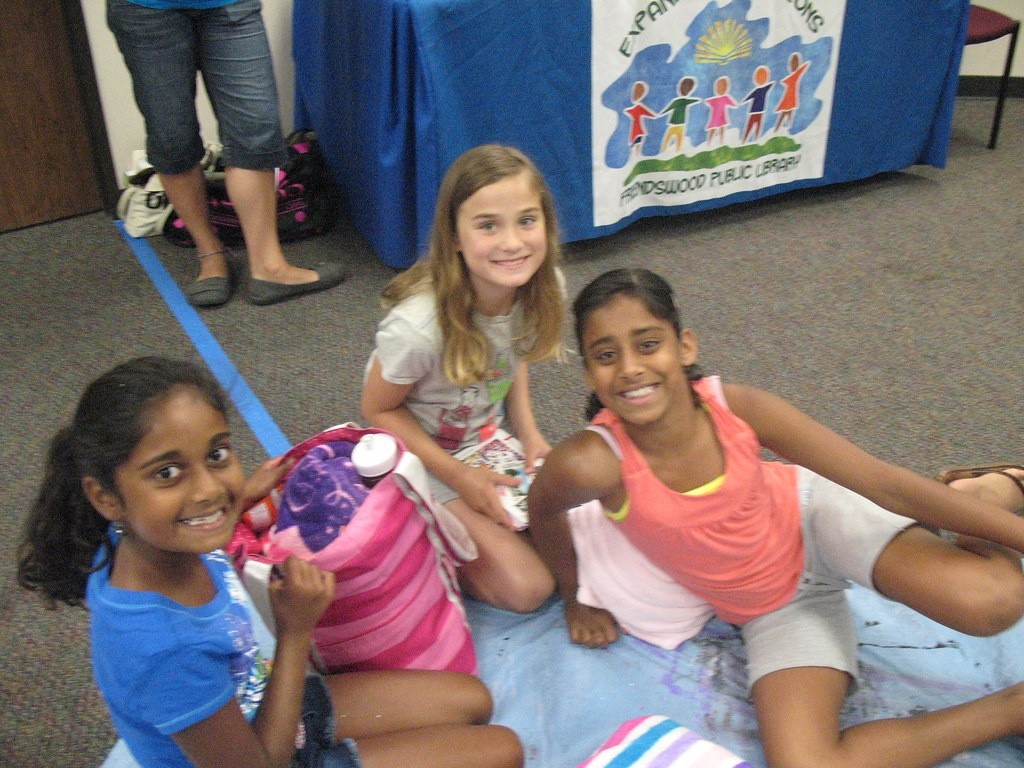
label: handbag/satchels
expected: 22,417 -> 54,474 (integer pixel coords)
222,420 -> 480,675
116,142 -> 223,238
164,128 -> 340,248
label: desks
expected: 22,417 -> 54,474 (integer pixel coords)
291,0 -> 970,275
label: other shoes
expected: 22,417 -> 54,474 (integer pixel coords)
249,262 -> 348,305
186,261 -> 235,305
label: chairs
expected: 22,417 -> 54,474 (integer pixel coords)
965,3 -> 1021,150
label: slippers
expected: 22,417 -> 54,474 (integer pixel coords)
944,463 -> 1024,496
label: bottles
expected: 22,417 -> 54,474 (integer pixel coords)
351,433 -> 399,489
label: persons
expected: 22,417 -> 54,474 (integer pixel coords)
105,0 -> 346,306
525,267 -> 1024,768
14,357 -> 525,768
360,146 -> 567,614
